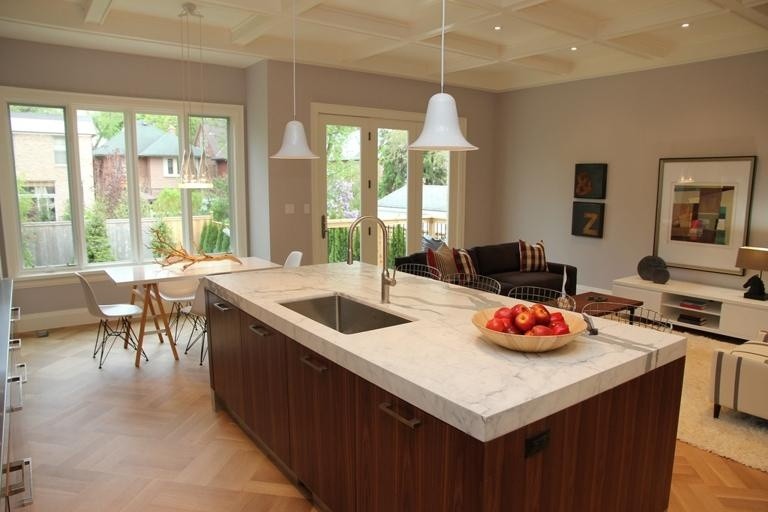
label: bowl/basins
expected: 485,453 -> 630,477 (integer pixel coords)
470,307 -> 587,354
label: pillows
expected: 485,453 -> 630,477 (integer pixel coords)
426,243 -> 478,284
518,238 -> 549,272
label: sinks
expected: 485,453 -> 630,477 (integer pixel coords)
274,290 -> 421,335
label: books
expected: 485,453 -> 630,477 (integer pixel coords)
680,298 -> 706,306
678,317 -> 708,326
679,303 -> 708,310
679,313 -> 707,321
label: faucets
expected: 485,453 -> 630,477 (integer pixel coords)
347,214 -> 398,304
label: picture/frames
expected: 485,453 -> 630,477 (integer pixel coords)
652,155 -> 758,276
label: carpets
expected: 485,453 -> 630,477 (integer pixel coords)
595,310 -> 768,475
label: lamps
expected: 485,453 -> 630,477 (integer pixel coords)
268,0 -> 319,161
405,1 -> 479,153
175,1 -> 216,189
734,245 -> 767,280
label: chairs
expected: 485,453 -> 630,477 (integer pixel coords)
75,249 -> 303,368
705,329 -> 768,421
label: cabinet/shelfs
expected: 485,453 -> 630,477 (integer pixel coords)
609,274 -> 768,341
1,277 -> 35,511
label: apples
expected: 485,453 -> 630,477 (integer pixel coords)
485,303 -> 570,336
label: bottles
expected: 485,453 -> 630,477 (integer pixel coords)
688,219 -> 704,242
714,206 -> 727,245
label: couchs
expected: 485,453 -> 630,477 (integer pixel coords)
395,241 -> 578,303
